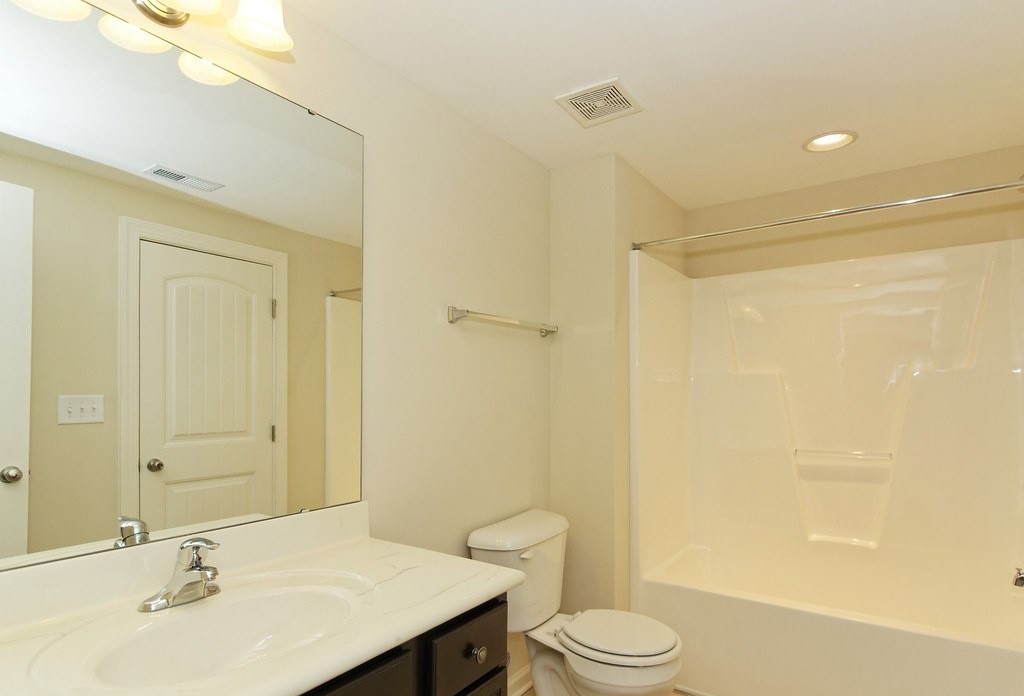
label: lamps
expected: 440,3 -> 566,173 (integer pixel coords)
12,0 -> 240,86
132,0 -> 293,53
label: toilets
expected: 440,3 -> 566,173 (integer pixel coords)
464,504 -> 683,694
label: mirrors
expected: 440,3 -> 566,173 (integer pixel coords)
0,0 -> 368,572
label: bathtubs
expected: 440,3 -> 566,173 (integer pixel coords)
603,542 -> 1024,693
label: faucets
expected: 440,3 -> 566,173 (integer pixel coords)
108,513 -> 151,547
138,537 -> 222,612
1012,568 -> 1024,587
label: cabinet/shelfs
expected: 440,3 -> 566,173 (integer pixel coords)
302,592 -> 509,696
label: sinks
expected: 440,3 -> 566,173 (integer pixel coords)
93,590 -> 354,689
